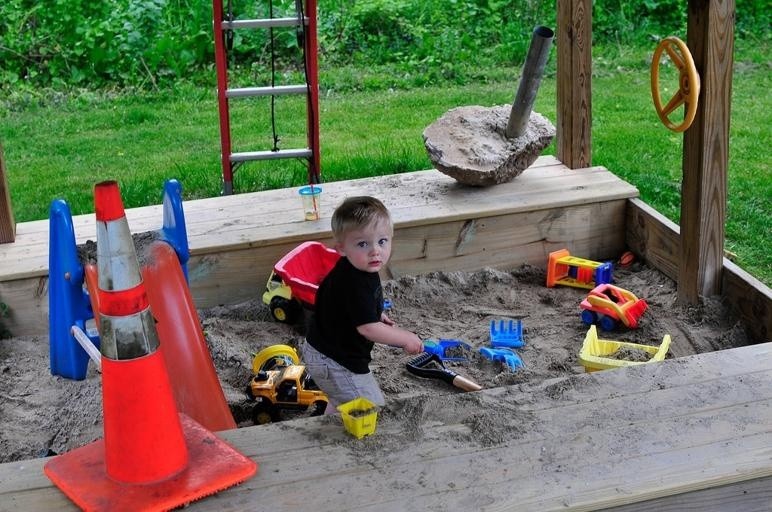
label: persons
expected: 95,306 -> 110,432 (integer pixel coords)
299,195 -> 426,416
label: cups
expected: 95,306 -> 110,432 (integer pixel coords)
299,187 -> 323,222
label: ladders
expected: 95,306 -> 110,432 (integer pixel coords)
213,0 -> 319,195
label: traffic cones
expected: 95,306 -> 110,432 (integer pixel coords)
44,178 -> 257,512
